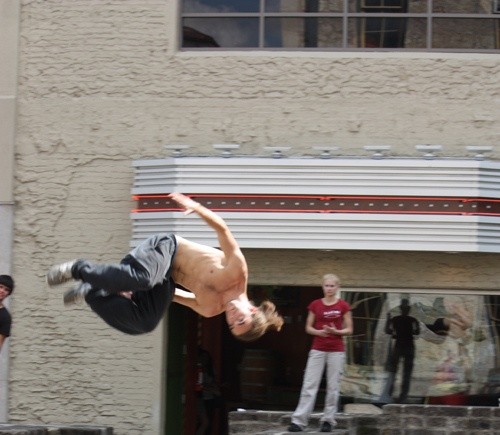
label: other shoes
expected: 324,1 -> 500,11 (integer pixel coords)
288,425 -> 301,432
320,422 -> 332,432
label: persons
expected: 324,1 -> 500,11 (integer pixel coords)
378,299 -> 420,406
0,274 -> 15,349
47,191 -> 284,343
287,274 -> 355,433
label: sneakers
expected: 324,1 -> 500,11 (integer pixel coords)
48,259 -> 78,286
65,283 -> 90,308
